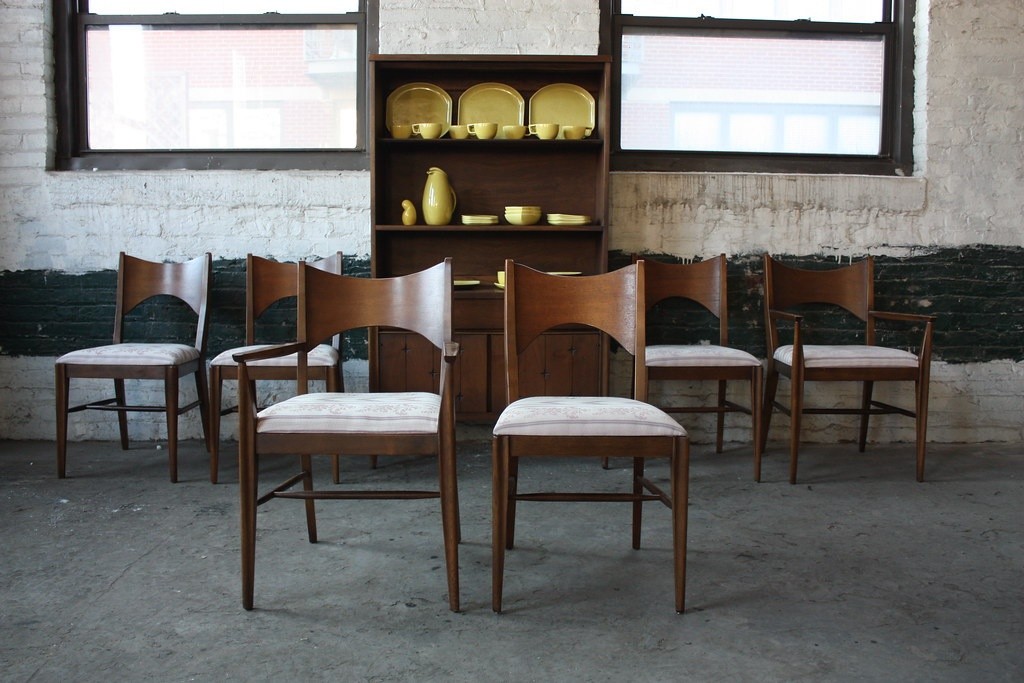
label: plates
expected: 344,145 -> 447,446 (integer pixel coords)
528,82 -> 596,139
546,213 -> 593,226
453,280 -> 482,287
385,82 -> 453,139
494,283 -> 505,290
456,83 -> 524,140
461,214 -> 499,225
547,272 -> 582,275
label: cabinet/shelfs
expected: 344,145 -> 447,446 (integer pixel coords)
363,55 -> 613,469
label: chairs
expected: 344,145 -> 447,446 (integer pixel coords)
491,259 -> 689,614
632,253 -> 764,483
762,254 -> 938,484
232,257 -> 461,612
211,251 -> 344,484
55,251 -> 209,484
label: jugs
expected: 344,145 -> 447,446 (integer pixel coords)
422,166 -> 456,226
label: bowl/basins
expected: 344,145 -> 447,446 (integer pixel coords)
497,271 -> 505,285
504,205 -> 542,225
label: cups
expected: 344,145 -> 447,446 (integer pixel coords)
528,122 -> 560,139
562,125 -> 593,139
503,125 -> 532,140
467,121 -> 498,140
411,122 -> 442,139
446,124 -> 468,139
